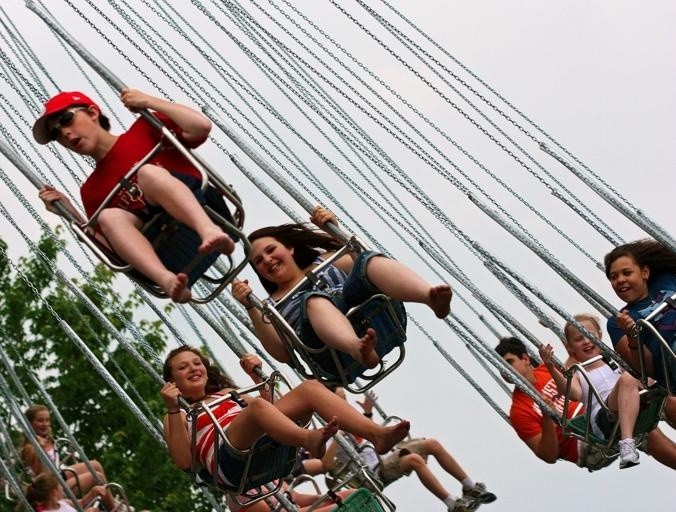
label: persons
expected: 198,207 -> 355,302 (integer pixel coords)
284,445 -> 326,489
326,386 -> 498,512
495,336 -> 676,471
539,315 -> 643,469
232,205 -> 452,369
33,88 -> 235,304
160,345 -> 410,494
225,478 -> 358,512
605,237 -> 676,397
15,403 -> 135,512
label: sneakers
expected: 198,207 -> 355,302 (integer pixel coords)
447,497 -> 479,511
461,481 -> 497,503
618,437 -> 639,468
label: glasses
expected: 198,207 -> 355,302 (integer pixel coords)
46,107 -> 88,141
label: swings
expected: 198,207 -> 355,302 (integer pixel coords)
56,262 -> 314,505
184,104 -> 408,395
419,190 -> 671,461
1,2 -> 256,305
466,139 -> 676,398
1,346 -> 133,512
196,362 -> 418,512
432,290 -> 624,473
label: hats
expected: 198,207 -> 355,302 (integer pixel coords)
32,91 -> 100,145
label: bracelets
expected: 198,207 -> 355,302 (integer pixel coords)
363,413 -> 372,418
168,410 -> 181,414
